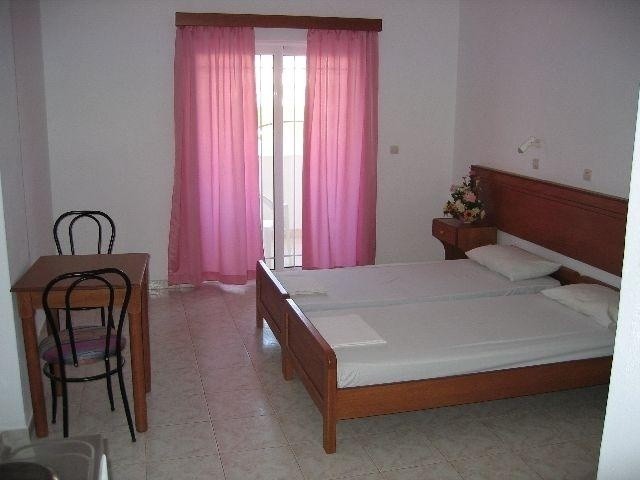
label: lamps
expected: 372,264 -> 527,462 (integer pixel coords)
518,135 -> 544,153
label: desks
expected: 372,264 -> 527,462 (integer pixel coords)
9,253 -> 151,437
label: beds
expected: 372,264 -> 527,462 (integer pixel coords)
284,276 -> 620,455
256,259 -> 580,377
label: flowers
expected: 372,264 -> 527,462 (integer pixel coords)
443,169 -> 488,223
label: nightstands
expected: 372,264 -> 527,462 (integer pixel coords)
432,218 -> 497,260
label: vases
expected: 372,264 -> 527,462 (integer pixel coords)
459,214 -> 472,224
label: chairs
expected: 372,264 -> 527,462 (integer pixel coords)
36,268 -> 137,443
52,211 -> 116,330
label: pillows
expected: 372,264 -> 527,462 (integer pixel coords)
464,244 -> 563,283
541,284 -> 620,331
